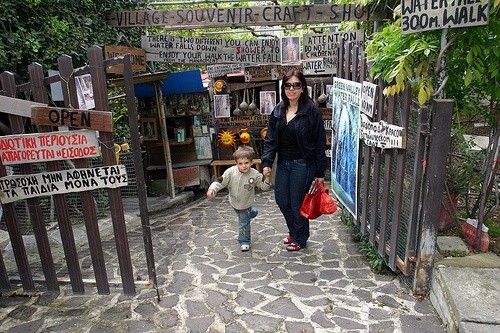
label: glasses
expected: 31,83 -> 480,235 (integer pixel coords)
284,82 -> 302,90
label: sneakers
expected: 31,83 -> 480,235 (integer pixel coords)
254,209 -> 258,217
241,244 -> 249,251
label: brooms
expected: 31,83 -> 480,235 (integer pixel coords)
463,204 -> 496,252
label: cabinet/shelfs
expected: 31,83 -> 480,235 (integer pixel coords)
140,58 -> 329,187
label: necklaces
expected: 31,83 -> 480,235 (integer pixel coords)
287,112 -> 295,119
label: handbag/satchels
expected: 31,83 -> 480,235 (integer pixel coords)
300,179 -> 329,219
316,178 -> 337,215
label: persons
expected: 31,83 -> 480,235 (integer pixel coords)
206,146 -> 270,252
262,70 -> 327,252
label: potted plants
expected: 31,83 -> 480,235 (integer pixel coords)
434,164 -> 480,232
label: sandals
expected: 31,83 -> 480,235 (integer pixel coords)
286,242 -> 302,251
284,236 -> 296,244
